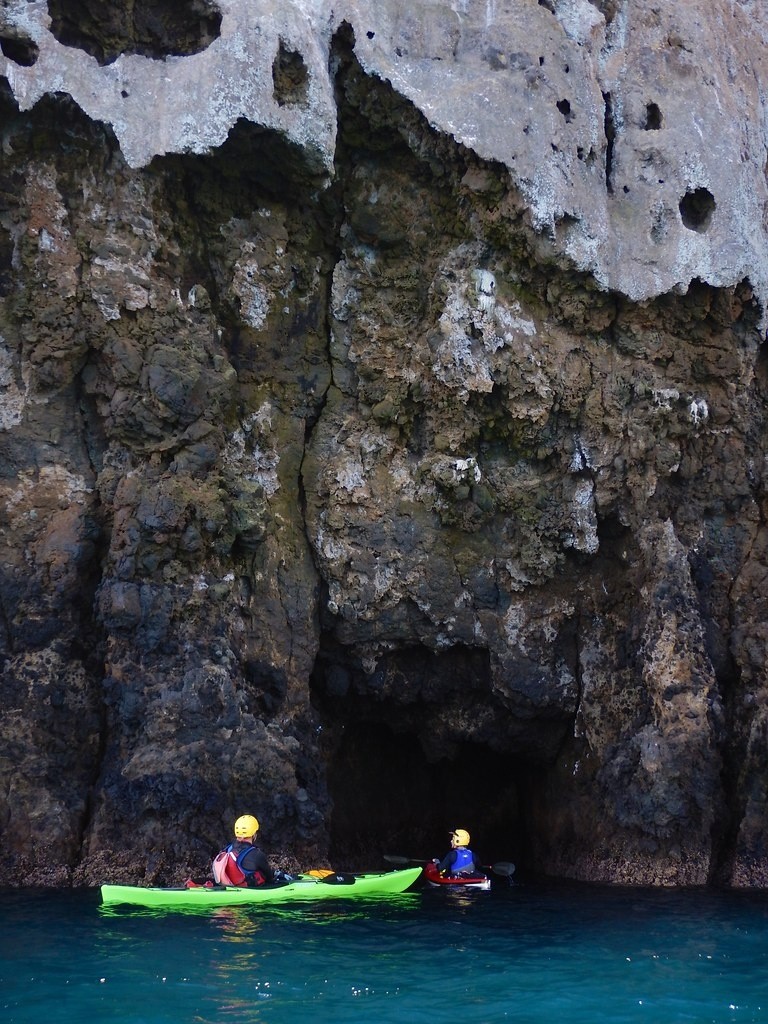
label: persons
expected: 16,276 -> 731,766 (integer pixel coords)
435,828 -> 484,877
212,814 -> 277,889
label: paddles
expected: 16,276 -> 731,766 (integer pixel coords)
274,873 -> 353,885
383,854 -> 515,876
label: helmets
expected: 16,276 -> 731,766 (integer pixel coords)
234,814 -> 260,838
453,828 -> 470,846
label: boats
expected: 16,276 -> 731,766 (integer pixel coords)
101,866 -> 423,906
426,864 -> 490,890
